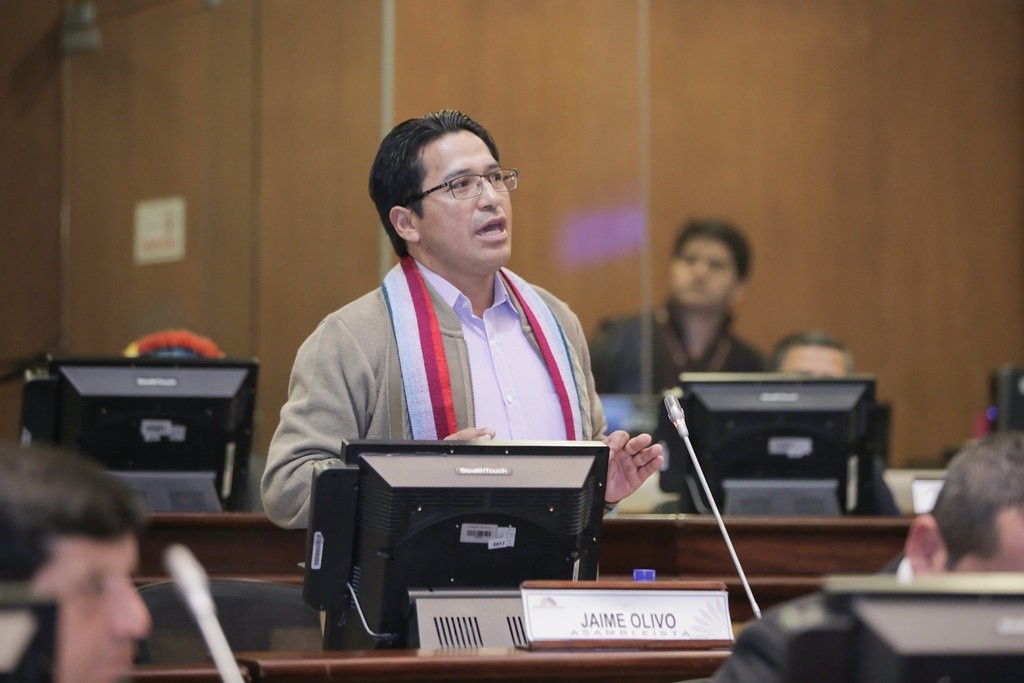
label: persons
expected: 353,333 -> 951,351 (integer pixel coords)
654,330 -> 902,517
0,446 -> 153,682
262,109 -> 665,635
590,219 -> 771,395
718,431 -> 1024,683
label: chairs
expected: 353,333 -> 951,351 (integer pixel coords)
133,577 -> 329,664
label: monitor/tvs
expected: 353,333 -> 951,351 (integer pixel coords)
306,440 -> 610,652
658,373 -> 879,517
779,573 -> 1024,683
18,355 -> 260,514
987,368 -> 1024,435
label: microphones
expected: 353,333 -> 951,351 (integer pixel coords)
160,542 -> 247,682
663,393 -> 760,619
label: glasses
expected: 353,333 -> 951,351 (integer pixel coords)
386,168 -> 519,208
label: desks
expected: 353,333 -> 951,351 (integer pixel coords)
119,514 -> 926,683
